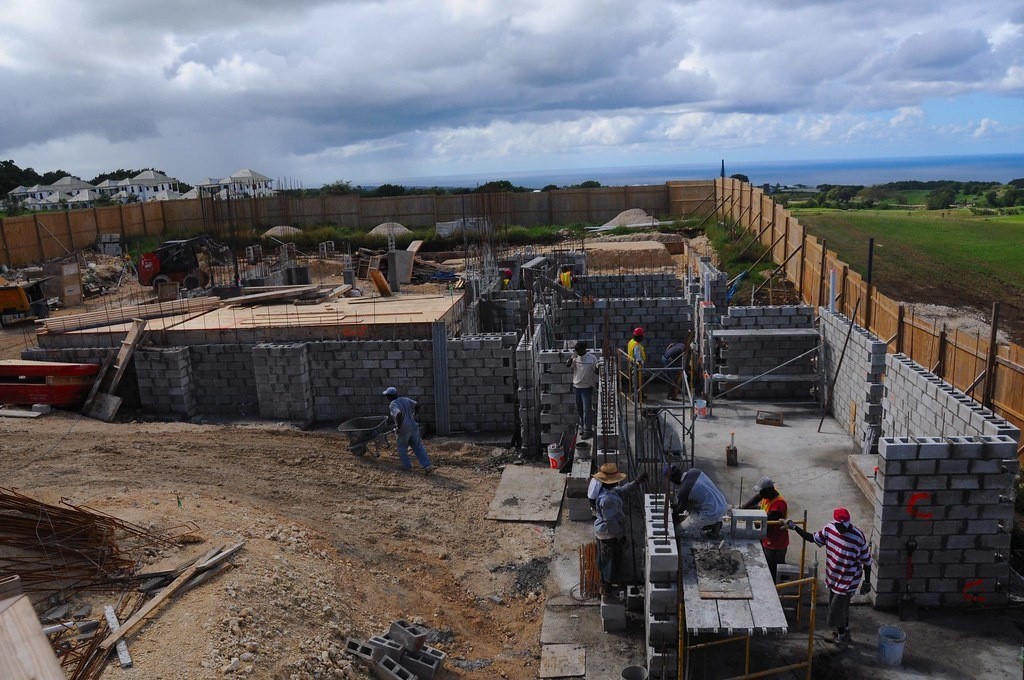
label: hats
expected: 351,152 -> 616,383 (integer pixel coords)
834,507 -> 850,523
663,464 -> 679,478
752,477 -> 774,491
383,387 -> 398,395
591,462 -> 626,484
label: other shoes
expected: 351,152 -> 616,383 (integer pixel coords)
707,522 -> 722,540
824,628 -> 851,643
599,582 -> 620,596
667,393 -> 681,400
829,637 -> 848,655
603,591 -> 626,604
579,425 -> 593,440
425,468 -> 432,475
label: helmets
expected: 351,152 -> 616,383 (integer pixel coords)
505,269 -> 511,275
633,327 -> 644,336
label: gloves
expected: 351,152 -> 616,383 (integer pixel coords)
779,518 -> 797,530
635,472 -> 648,483
412,413 -> 417,419
570,351 -> 579,359
618,536 -> 626,545
596,361 -> 604,369
395,426 -> 402,435
860,581 -> 871,595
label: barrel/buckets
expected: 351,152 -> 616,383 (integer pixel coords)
878,626 -> 907,666
694,400 -> 707,418
575,442 -> 591,458
547,444 -> 564,470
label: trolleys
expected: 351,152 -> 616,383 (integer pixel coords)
338,415 -> 398,454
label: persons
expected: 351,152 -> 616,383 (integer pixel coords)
627,327 -> 647,402
588,472 -> 651,517
663,464 -> 728,537
661,342 -> 687,401
558,264 -> 578,291
381,387 -> 434,476
778,508 -> 871,646
735,477 -> 791,583
568,341 -> 601,440
594,462 -> 626,600
